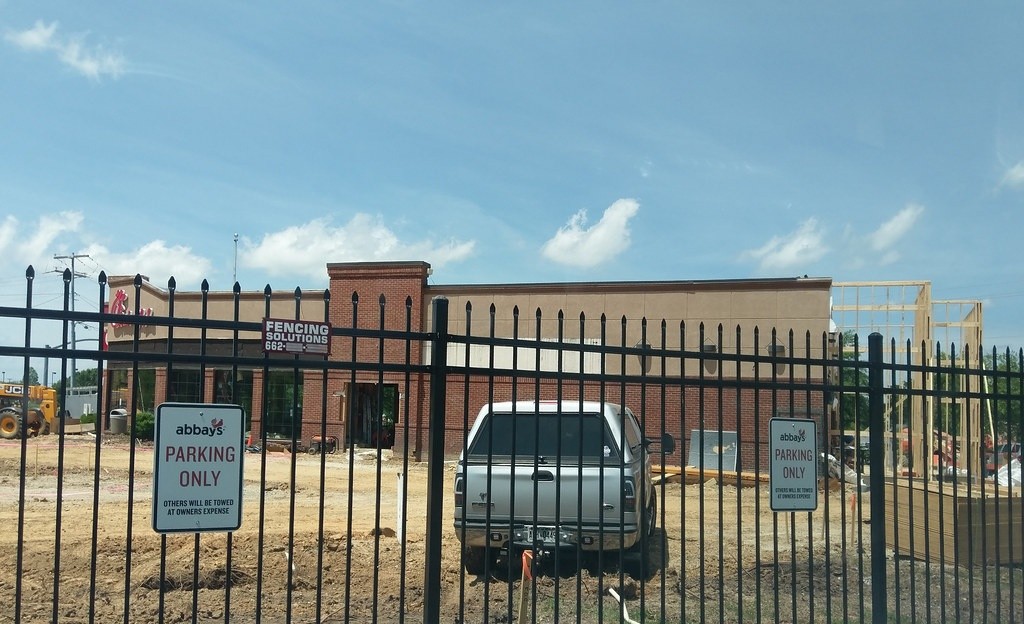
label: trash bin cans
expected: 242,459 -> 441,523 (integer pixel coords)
110,409 -> 128,435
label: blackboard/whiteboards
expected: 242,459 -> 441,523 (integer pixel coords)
689,430 -> 737,472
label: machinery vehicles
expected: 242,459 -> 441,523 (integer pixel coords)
899,426 -> 1021,480
0,382 -> 56,438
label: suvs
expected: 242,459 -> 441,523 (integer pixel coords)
454,399 -> 677,576
